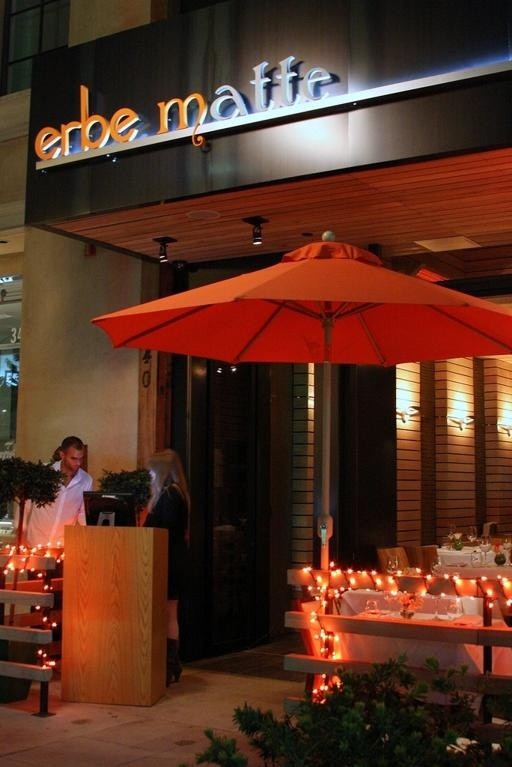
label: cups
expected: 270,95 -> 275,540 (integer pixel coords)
383,553 -> 444,578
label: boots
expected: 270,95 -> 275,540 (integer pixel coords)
166,638 -> 181,686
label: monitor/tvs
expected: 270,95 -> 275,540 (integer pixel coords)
83,490 -> 136,527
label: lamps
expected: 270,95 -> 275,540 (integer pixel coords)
152,232 -> 176,262
242,217 -> 270,247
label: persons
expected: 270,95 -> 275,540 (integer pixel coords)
140,447 -> 193,687
12,435 -> 94,671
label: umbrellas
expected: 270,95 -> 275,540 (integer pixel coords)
88,229 -> 512,570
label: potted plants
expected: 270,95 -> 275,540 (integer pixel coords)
1,457 -> 64,702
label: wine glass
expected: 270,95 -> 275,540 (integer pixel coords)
364,589 -> 459,621
445,524 -> 512,568
